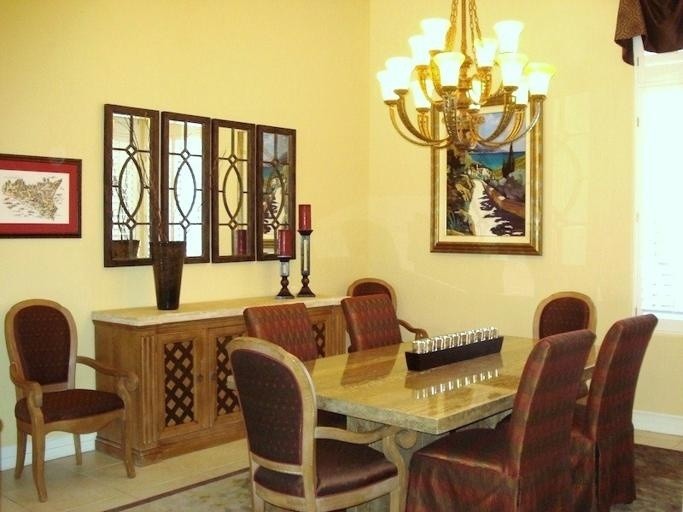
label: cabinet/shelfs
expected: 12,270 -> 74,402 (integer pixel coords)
91,298 -> 355,467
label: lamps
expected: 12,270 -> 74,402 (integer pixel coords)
376,0 -> 548,152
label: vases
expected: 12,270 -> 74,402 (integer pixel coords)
112,239 -> 139,260
149,240 -> 186,310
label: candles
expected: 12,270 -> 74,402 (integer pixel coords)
278,230 -> 289,256
235,229 -> 247,255
298,204 -> 312,230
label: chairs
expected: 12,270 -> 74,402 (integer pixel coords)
532,292 -> 597,340
346,278 -> 430,344
340,293 -> 402,352
496,313 -> 659,511
4,298 -> 136,503
225,336 -> 419,511
405,329 -> 597,509
242,302 -> 317,363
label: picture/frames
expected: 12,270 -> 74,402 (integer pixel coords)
430,94 -> 544,256
0,153 -> 82,239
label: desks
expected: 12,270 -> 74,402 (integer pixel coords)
226,338 -> 597,511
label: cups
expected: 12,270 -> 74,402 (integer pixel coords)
410,368 -> 501,401
411,326 -> 499,353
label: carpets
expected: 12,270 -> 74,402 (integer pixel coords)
105,440 -> 681,511
